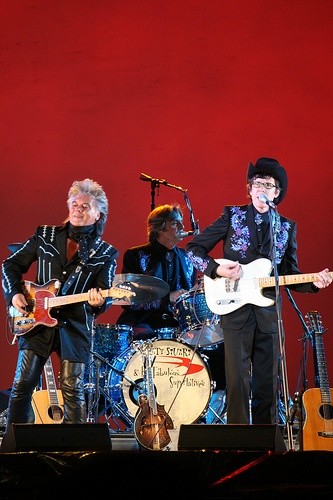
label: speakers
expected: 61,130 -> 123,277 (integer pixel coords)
0,423 -> 112,453
177,423 -> 288,451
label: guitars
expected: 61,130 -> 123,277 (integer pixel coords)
301,311 -> 333,452
203,257 -> 333,315
133,339 -> 174,451
30,356 -> 65,424
6,279 -> 137,336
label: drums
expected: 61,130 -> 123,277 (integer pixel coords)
107,337 -> 214,430
83,323 -> 134,395
157,327 -> 220,350
174,288 -> 225,347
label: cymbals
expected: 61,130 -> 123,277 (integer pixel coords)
112,273 -> 171,306
8,243 -> 21,251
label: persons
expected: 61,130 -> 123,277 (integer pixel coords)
113,204 -> 198,336
185,157 -> 332,425
0,179 -> 119,433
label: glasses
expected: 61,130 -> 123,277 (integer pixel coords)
251,182 -> 278,189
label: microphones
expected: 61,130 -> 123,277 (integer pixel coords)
258,194 -> 277,208
175,230 -> 195,238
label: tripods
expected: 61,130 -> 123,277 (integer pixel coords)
84,316 -> 133,431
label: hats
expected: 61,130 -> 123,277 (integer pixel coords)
248,157 -> 286,202
